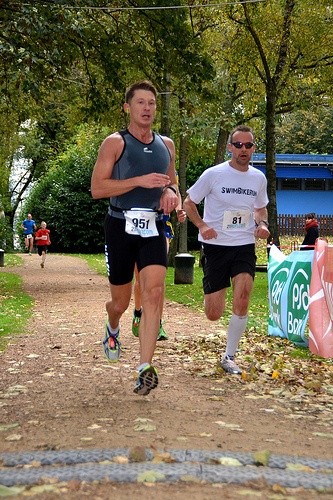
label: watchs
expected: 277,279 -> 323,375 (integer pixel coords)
259,220 -> 269,225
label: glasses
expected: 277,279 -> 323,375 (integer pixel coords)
231,142 -> 254,149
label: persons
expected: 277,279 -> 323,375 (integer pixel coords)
35,221 -> 51,268
21,214 -> 37,256
90,81 -> 180,396
300,213 -> 319,251
132,169 -> 187,341
183,126 -> 269,374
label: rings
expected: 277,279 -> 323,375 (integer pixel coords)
172,203 -> 176,205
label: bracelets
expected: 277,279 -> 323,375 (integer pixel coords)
167,186 -> 177,195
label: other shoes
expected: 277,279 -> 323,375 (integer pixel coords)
41,264 -> 44,268
29,252 -> 32,256
25,247 -> 28,250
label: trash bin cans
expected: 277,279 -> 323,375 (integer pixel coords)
0,249 -> 5,267
174,252 -> 195,284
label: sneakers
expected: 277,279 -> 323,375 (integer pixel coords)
220,355 -> 242,375
133,365 -> 159,396
132,308 -> 143,337
157,324 -> 167,341
102,319 -> 121,363
